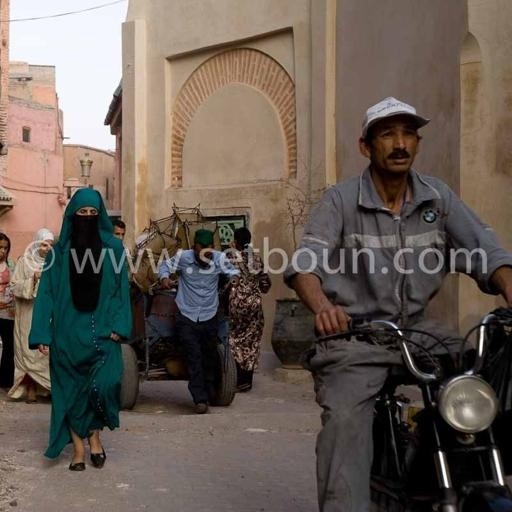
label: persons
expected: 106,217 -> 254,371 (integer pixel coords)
158,230 -> 240,414
284,98 -> 510,512
7,228 -> 54,405
223,226 -> 271,390
110,218 -> 127,241
29,188 -> 133,471
1,233 -> 18,388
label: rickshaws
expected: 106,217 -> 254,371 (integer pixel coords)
120,273 -> 238,411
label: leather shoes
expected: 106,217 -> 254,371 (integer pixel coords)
195,402 -> 207,414
90,447 -> 106,469
68,463 -> 85,472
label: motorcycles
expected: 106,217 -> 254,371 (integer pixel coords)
315,307 -> 512,511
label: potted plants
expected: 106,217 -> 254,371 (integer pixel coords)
271,175 -> 315,369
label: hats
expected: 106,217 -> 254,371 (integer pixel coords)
362,96 -> 431,139
195,229 -> 214,246
